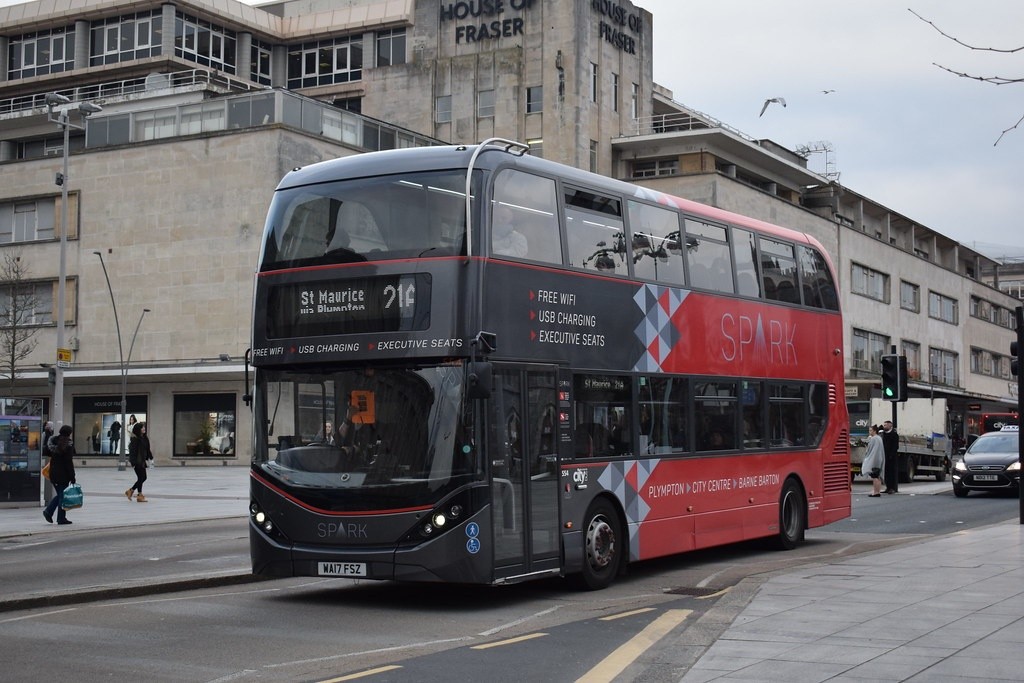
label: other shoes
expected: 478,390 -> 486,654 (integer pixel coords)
888,489 -> 897,494
42,510 -> 54,523
880,488 -> 889,493
137,494 -> 149,502
125,488 -> 134,501
57,517 -> 72,524
868,493 -> 881,497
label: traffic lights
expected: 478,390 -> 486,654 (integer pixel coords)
1007,306 -> 1022,376
880,354 -> 900,401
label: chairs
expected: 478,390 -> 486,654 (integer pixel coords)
672,257 -> 835,310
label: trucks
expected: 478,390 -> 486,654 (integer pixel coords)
870,397 -> 953,484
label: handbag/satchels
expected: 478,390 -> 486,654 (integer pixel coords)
146,458 -> 155,470
61,481 -> 83,511
41,457 -> 52,479
869,467 -> 881,478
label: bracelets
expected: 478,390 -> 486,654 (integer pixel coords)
343,418 -> 352,425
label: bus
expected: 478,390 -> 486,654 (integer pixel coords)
846,401 -> 872,482
244,137 -> 850,593
979,413 -> 1020,437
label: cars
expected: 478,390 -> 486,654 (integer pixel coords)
952,425 -> 1021,498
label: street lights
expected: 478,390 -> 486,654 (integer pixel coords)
46,92 -> 102,438
93,251 -> 151,471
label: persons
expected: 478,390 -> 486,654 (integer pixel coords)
491,206 -> 528,258
41,420 -> 76,524
109,421 -> 121,454
861,421 -> 899,497
125,423 -> 153,502
315,420 -> 334,445
127,414 -> 138,441
334,373 -> 383,455
12,423 -> 20,440
92,421 -> 100,452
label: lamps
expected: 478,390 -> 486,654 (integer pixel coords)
220,352 -> 231,361
